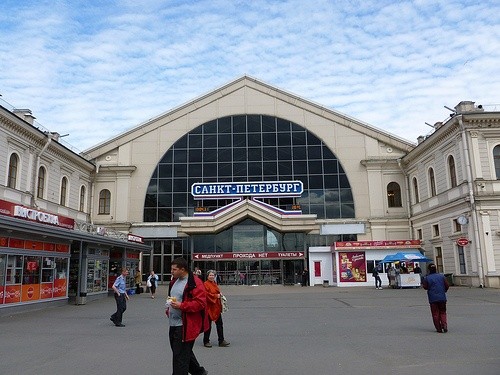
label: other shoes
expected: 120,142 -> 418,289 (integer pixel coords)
437,330 -> 442,333
444,329 -> 447,332
115,323 -> 126,327
110,316 -> 115,324
219,341 -> 230,346
376,287 -> 382,290
152,296 -> 154,299
204,342 -> 212,347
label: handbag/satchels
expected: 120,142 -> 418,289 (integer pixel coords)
221,294 -> 229,314
147,280 -> 152,288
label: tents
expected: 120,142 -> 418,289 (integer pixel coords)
380,252 -> 433,263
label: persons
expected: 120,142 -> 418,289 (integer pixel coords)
386,263 -> 408,287
147,271 -> 159,299
134,269 -> 141,288
423,263 -> 450,332
202,269 -> 232,348
109,268 -> 129,327
373,264 -> 383,290
164,257 -> 211,375
413,263 -> 422,275
301,267 -> 309,287
192,266 -> 202,279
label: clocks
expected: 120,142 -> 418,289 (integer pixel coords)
457,216 -> 467,225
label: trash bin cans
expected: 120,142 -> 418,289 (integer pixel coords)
76,292 -> 87,305
323,280 -> 329,288
